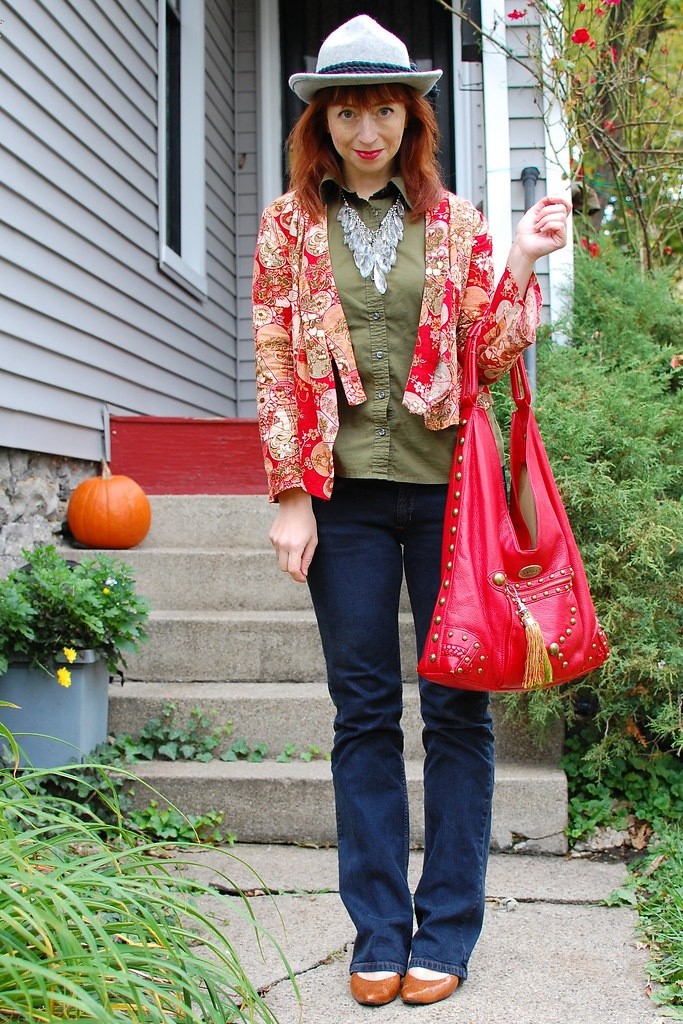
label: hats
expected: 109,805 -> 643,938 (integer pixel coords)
288,13 -> 441,104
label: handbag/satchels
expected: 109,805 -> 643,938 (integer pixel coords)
415,312 -> 608,691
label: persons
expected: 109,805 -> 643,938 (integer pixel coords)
251,11 -> 610,1010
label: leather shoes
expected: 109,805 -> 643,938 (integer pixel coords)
399,966 -> 459,1003
347,971 -> 400,1005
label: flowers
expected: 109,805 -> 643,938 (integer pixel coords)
2,539 -> 148,687
445,0 -> 681,382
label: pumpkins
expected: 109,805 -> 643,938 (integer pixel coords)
67,459 -> 152,549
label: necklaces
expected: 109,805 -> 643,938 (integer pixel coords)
336,185 -> 404,294
